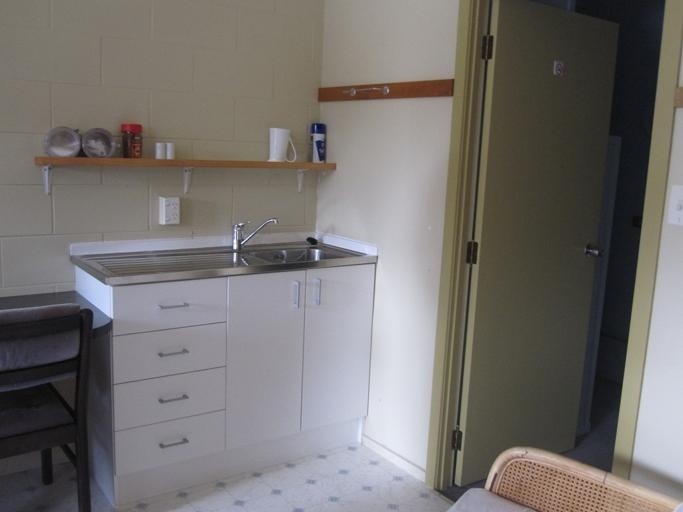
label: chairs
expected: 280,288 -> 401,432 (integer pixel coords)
0,301 -> 93,512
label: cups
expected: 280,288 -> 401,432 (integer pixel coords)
153,142 -> 179,160
263,126 -> 297,163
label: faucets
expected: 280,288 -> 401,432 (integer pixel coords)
231,216 -> 280,250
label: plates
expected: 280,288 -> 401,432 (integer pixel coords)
42,126 -> 115,160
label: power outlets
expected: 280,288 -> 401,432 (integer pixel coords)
157,194 -> 180,226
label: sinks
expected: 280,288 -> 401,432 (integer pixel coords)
243,241 -> 365,265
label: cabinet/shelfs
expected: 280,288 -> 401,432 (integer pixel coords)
223,262 -> 377,480
72,263 -> 227,507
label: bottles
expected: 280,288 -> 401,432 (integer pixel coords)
308,123 -> 329,163
121,122 -> 143,158
